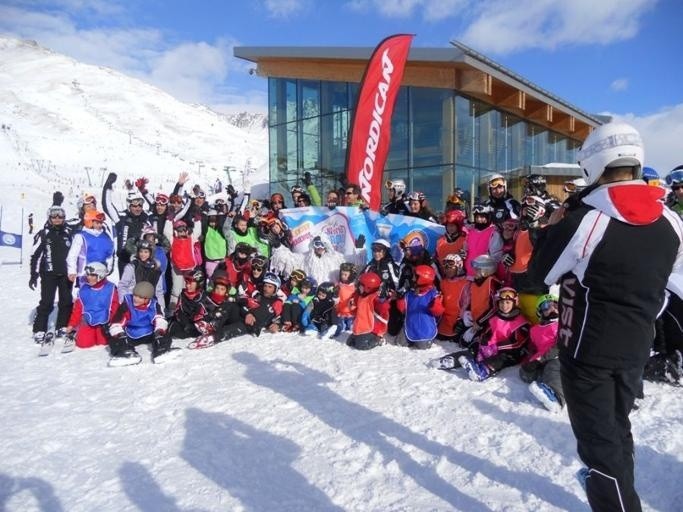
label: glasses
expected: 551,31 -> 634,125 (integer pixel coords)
345,193 -> 363,196
314,241 -> 326,249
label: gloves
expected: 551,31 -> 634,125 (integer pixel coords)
125,177 -> 148,189
500,253 -> 513,268
273,239 -> 281,248
325,199 -> 389,216
354,234 -> 367,248
52,189 -> 64,202
105,172 -> 117,190
461,310 -> 479,342
225,184 -> 257,199
29,272 -> 39,291
166,213 -> 206,220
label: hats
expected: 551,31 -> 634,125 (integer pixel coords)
270,192 -> 284,209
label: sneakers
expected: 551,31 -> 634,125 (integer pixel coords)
645,350 -> 682,381
305,323 -> 339,339
530,381 -> 561,412
34,327 -> 75,343
152,346 -> 183,362
267,321 -> 302,336
187,319 -> 214,349
459,355 -> 489,381
432,354 -> 458,370
107,353 -> 143,367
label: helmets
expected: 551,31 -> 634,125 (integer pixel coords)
84,261 -> 109,286
76,193 -> 105,230
208,199 -> 282,232
384,173 -> 547,235
290,185 -> 311,204
576,122 -> 644,181
184,269 -> 230,296
640,162 -> 683,186
413,264 -> 437,289
371,238 -> 424,255
494,288 -> 521,316
46,205 -> 65,227
133,225 -> 157,301
289,269 -> 334,299
535,294 -> 560,323
340,262 -> 384,294
174,220 -> 189,237
234,241 -> 281,295
126,186 -> 205,215
441,255 -> 494,281
564,178 -> 587,197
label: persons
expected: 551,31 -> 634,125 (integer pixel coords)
526,122 -> 683,512
28,164 -> 683,412
